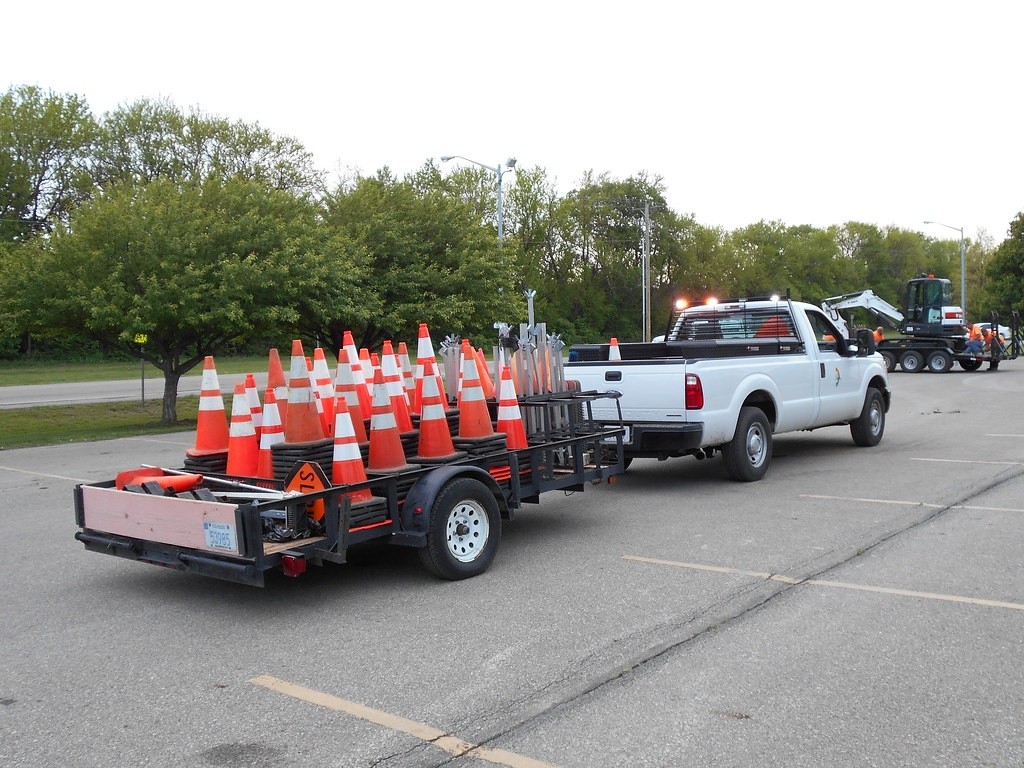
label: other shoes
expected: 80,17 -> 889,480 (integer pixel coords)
987,367 -> 997,371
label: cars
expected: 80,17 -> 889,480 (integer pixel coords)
973,320 -> 1011,340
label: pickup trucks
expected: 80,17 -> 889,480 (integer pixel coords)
550,299 -> 891,484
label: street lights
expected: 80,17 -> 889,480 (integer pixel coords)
441,155 -> 518,293
923,220 -> 966,320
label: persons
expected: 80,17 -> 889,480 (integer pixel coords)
932,285 -> 949,303
822,330 -> 836,341
960,322 -> 983,355
981,327 -> 1004,372
873,326 -> 884,347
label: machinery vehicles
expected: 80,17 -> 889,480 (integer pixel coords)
820,273 -> 1024,375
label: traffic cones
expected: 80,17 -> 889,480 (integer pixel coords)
116,466 -> 202,497
181,322 -> 531,529
608,336 -> 622,361
